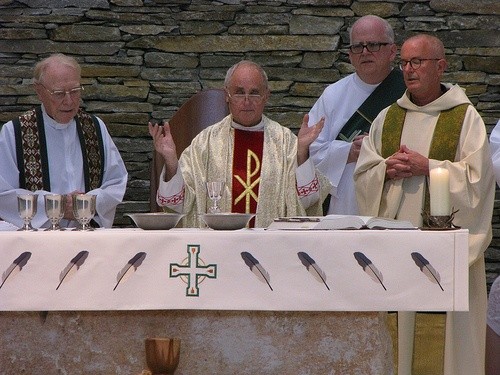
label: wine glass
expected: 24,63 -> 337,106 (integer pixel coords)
16,193 -> 40,231
71,193 -> 97,231
43,194 -> 68,231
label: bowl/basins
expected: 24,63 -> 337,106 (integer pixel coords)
123,212 -> 186,230
202,213 -> 254,230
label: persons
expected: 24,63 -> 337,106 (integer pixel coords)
486,118 -> 500,375
353,35 -> 496,375
0,53 -> 129,230
147,61 -> 326,228
305,14 -> 407,215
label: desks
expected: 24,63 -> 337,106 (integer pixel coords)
0,230 -> 469,375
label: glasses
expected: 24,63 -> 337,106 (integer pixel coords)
38,82 -> 84,99
397,57 -> 442,71
225,88 -> 266,104
349,41 -> 394,54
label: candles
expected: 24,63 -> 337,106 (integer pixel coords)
430,167 -> 451,216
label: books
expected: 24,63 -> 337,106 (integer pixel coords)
264,215 -> 419,231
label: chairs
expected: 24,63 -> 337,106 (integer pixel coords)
150,89 -> 230,214
